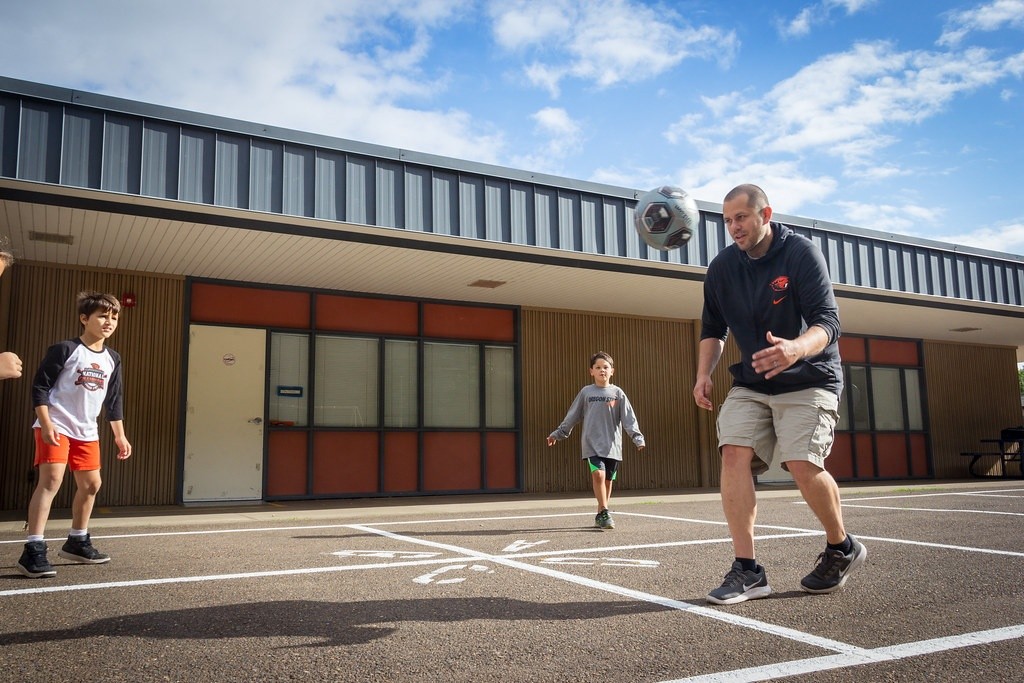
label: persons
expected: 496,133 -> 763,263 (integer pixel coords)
0,236 -> 23,380
547,352 -> 645,529
16,294 -> 132,578
693,184 -> 867,605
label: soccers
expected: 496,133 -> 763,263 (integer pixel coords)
634,185 -> 700,251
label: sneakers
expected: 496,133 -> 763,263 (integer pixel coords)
706,562 -> 771,604
16,541 -> 57,578
594,513 -> 602,527
800,533 -> 867,593
58,533 -> 111,564
600,509 -> 615,529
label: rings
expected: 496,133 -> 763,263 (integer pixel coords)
774,361 -> 778,367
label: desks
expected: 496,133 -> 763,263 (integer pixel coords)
980,438 -> 1024,480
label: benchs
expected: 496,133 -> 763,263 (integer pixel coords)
961,451 -> 1020,480
